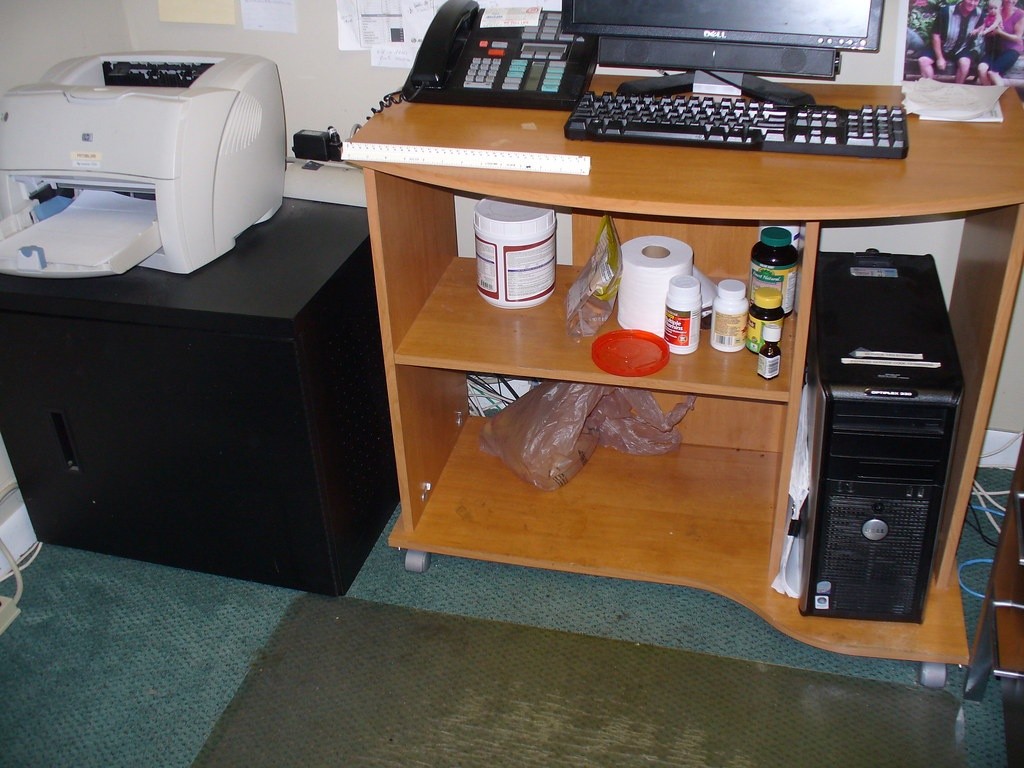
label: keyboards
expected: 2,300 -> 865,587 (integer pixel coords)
565,89 -> 910,158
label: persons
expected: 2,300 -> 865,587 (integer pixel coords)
906,0 -> 1024,87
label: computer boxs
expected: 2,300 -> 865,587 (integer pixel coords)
797,249 -> 964,620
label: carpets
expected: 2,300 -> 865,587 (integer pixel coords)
190,595 -> 968,768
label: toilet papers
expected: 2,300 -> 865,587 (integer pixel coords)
616,235 -> 693,340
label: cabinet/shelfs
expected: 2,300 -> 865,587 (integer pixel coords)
344,71 -> 1024,688
0,196 -> 400,598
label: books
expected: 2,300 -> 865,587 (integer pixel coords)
919,100 -> 1004,123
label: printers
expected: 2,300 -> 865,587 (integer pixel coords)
0,49 -> 284,278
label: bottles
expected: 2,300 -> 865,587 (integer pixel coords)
747,227 -> 800,318
757,324 -> 782,380
472,196 -> 559,309
745,287 -> 785,355
710,279 -> 750,352
664,276 -> 701,355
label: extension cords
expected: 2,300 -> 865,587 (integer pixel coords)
283,157 -> 368,208
0,596 -> 20,634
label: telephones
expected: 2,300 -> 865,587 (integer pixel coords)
399,0 -> 598,111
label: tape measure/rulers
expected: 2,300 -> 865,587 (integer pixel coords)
341,141 -> 591,176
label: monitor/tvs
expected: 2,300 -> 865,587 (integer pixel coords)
562,0 -> 885,105
